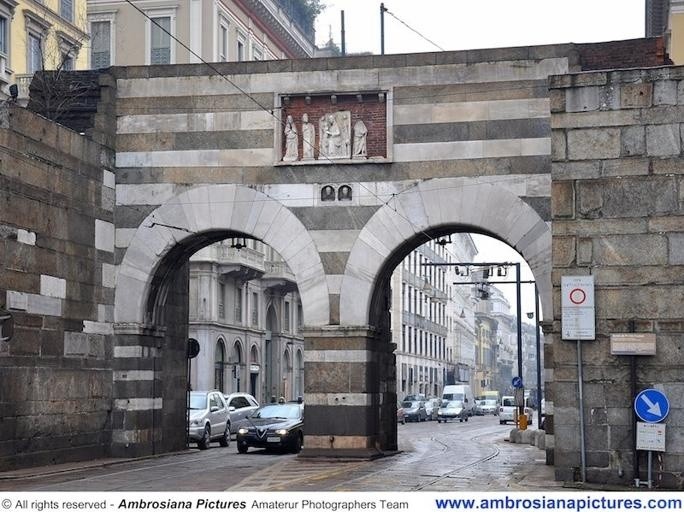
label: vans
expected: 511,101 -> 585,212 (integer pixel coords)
438,385 -> 475,416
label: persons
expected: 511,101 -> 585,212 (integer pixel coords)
302,113 -> 316,159
352,120 -> 368,156
282,115 -> 299,161
323,115 -> 341,156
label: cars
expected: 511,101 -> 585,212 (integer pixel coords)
475,391 -> 533,424
186,389 -> 304,454
397,394 -> 468,424
